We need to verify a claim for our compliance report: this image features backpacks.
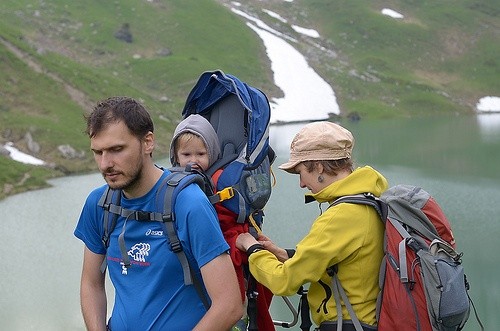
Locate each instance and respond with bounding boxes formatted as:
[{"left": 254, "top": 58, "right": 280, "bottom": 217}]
[{"left": 305, "top": 183, "right": 472, "bottom": 331}]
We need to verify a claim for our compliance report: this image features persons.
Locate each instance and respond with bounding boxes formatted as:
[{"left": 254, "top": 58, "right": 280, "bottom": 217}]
[
  {"left": 73, "top": 96, "right": 243, "bottom": 330},
  {"left": 235, "top": 119, "right": 404, "bottom": 331},
  {"left": 171, "top": 113, "right": 263, "bottom": 297}
]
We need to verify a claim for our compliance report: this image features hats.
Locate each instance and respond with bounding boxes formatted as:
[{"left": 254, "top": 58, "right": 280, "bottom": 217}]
[{"left": 277, "top": 121, "right": 355, "bottom": 174}]
